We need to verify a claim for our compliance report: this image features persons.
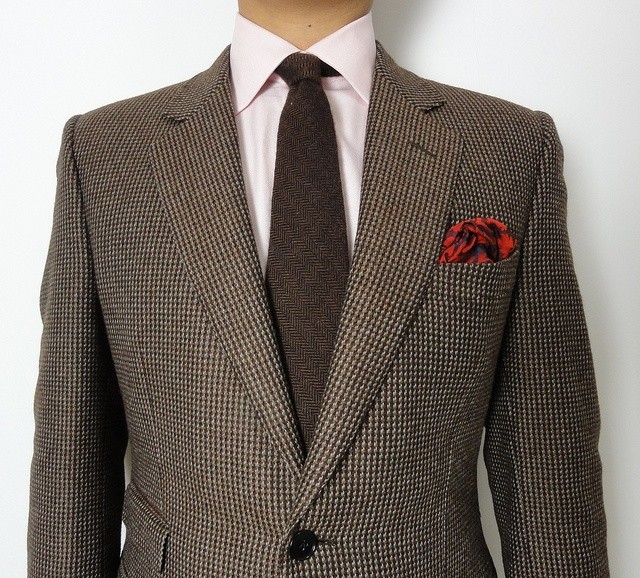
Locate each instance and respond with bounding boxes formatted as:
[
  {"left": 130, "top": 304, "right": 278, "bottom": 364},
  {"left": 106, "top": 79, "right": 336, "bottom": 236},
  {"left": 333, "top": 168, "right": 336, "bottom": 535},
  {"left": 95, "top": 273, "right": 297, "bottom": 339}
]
[{"left": 26, "top": 1, "right": 611, "bottom": 577}]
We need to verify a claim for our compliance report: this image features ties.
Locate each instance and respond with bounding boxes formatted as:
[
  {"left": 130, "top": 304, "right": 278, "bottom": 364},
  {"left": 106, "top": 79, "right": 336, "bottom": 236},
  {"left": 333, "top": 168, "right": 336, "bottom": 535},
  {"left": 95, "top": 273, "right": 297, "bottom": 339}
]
[{"left": 265, "top": 53, "right": 349, "bottom": 458}]
